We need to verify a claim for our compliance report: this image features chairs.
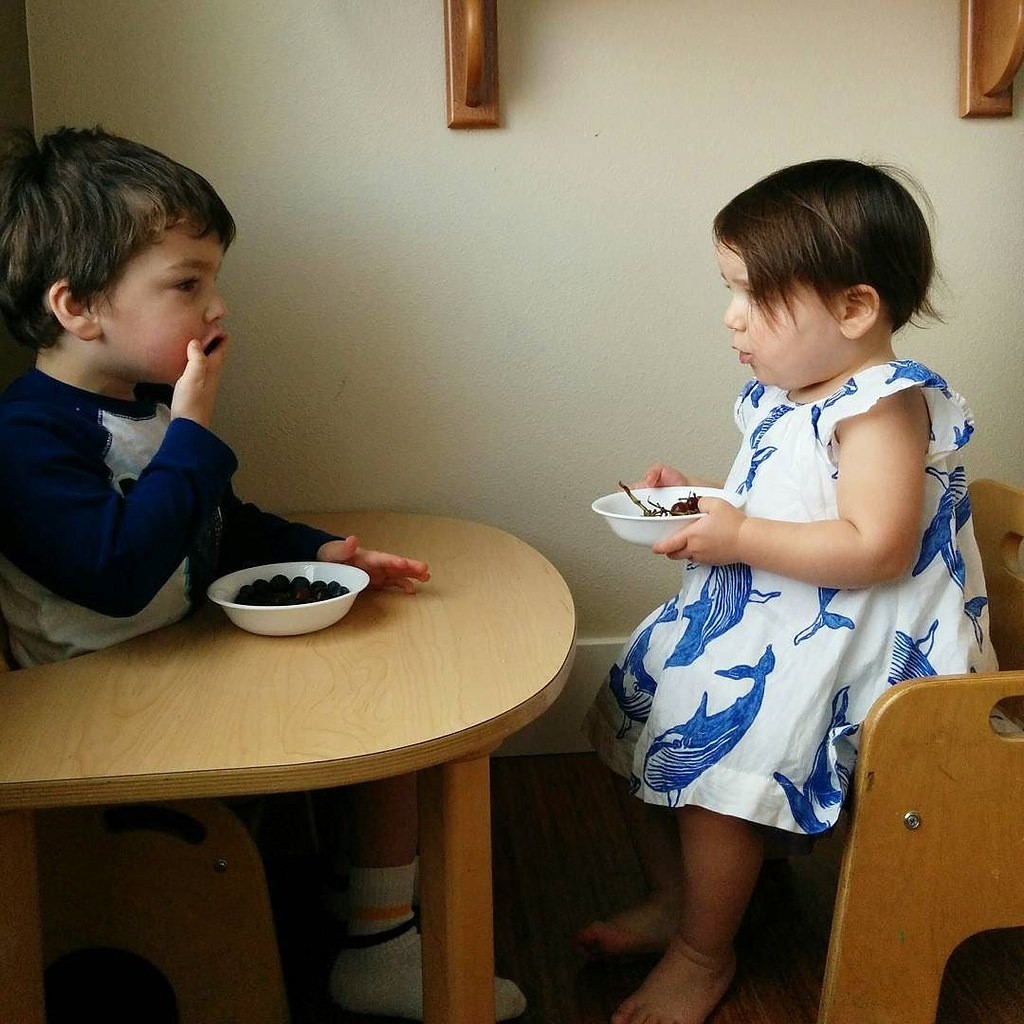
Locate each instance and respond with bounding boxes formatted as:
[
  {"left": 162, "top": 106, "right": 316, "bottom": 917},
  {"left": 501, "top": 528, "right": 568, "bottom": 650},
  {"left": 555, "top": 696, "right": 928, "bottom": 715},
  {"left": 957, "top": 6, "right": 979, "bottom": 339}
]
[{"left": 814, "top": 484, "right": 1023, "bottom": 1024}]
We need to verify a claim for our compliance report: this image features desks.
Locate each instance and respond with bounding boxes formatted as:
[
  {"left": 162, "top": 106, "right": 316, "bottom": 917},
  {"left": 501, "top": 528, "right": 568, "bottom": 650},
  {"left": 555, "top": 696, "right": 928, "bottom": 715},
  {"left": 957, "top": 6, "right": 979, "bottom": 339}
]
[{"left": 0, "top": 513, "right": 584, "bottom": 1024}]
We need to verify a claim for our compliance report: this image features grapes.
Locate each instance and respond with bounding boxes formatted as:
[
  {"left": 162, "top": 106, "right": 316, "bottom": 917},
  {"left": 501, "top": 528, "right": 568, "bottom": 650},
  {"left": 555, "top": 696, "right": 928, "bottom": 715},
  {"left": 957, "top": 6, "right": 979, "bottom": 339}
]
[
  {"left": 234, "top": 573, "right": 349, "bottom": 606},
  {"left": 670, "top": 497, "right": 699, "bottom": 516}
]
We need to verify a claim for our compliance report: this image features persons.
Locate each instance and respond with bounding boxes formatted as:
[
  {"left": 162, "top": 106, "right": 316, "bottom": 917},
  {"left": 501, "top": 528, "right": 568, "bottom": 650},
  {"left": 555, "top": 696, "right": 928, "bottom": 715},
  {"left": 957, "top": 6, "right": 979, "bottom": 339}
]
[
  {"left": 580, "top": 155, "right": 1002, "bottom": 1024},
  {"left": 0, "top": 121, "right": 527, "bottom": 1023}
]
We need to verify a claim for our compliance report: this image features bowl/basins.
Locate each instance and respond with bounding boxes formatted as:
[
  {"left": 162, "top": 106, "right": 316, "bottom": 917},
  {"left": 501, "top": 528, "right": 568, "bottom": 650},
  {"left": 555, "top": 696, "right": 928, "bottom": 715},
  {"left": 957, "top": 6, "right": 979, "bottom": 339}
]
[
  {"left": 590, "top": 486, "right": 747, "bottom": 546},
  {"left": 207, "top": 561, "right": 371, "bottom": 635}
]
